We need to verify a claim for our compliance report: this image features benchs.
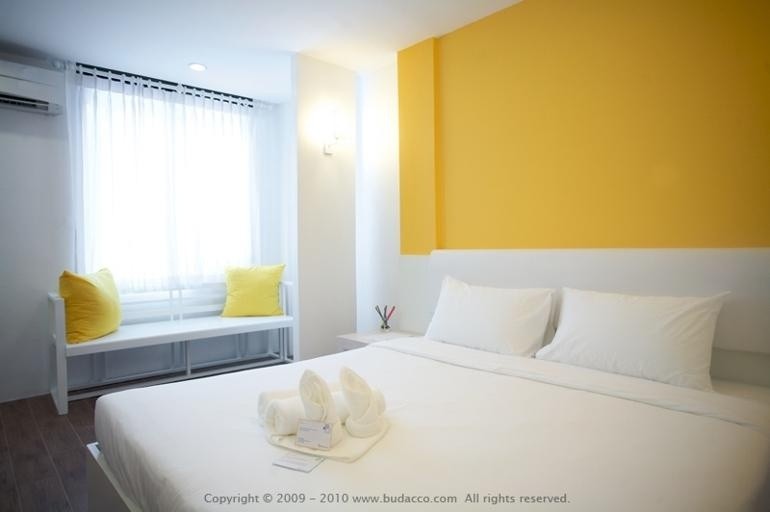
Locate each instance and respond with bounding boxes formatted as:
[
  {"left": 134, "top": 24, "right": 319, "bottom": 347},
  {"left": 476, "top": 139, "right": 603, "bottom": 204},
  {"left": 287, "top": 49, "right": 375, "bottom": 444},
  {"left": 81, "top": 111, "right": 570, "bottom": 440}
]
[{"left": 46, "top": 272, "right": 294, "bottom": 415}]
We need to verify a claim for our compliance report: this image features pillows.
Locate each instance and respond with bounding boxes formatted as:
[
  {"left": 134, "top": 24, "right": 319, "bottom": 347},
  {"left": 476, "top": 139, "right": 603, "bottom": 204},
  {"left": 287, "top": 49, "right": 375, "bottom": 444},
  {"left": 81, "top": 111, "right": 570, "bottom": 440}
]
[
  {"left": 219, "top": 262, "right": 284, "bottom": 317},
  {"left": 425, "top": 275, "right": 556, "bottom": 359},
  {"left": 537, "top": 286, "right": 730, "bottom": 397},
  {"left": 58, "top": 267, "right": 122, "bottom": 345}
]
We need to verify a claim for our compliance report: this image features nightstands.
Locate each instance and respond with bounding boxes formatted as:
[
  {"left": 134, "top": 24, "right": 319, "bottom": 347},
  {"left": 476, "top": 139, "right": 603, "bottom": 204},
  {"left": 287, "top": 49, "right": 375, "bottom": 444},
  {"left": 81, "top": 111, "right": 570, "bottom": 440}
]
[{"left": 335, "top": 330, "right": 413, "bottom": 356}]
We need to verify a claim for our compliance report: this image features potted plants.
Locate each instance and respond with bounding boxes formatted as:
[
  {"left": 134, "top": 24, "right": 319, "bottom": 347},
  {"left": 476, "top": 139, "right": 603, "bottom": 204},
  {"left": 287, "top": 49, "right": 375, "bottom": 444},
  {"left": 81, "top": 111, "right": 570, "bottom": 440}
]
[{"left": 373, "top": 304, "right": 395, "bottom": 337}]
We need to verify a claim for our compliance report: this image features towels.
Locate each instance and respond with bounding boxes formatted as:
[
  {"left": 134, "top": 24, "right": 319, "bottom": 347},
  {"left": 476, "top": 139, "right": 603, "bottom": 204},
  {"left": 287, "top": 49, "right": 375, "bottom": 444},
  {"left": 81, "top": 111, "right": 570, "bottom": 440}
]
[{"left": 252, "top": 355, "right": 396, "bottom": 469}]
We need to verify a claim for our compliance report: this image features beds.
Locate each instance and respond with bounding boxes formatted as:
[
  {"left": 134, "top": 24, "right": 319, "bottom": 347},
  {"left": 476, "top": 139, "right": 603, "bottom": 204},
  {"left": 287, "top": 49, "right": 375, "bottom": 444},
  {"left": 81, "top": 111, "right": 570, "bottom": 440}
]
[{"left": 79, "top": 242, "right": 770, "bottom": 512}]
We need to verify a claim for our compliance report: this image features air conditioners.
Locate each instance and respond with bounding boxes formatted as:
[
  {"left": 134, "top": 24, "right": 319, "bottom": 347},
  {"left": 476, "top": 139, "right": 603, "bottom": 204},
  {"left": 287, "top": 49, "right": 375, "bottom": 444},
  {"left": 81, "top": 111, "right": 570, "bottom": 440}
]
[{"left": 0, "top": 57, "right": 65, "bottom": 117}]
